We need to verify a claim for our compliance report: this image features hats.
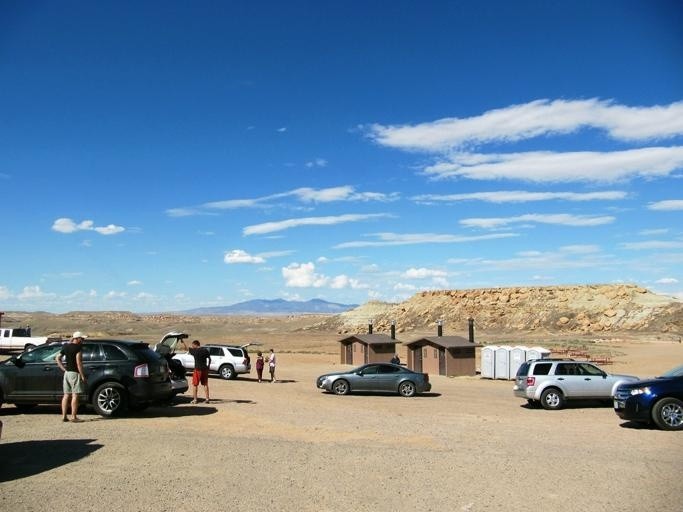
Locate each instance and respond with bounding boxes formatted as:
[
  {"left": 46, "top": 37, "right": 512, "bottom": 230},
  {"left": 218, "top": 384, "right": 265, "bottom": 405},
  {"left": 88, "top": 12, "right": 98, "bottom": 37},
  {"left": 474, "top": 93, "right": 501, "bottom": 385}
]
[{"left": 72, "top": 332, "right": 88, "bottom": 338}]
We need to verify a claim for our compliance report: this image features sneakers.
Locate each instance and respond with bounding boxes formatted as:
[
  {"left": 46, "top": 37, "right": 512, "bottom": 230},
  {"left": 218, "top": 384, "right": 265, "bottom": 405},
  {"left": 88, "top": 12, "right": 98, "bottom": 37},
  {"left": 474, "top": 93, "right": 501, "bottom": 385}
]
[
  {"left": 203, "top": 400, "right": 209, "bottom": 404},
  {"left": 191, "top": 400, "right": 198, "bottom": 404}
]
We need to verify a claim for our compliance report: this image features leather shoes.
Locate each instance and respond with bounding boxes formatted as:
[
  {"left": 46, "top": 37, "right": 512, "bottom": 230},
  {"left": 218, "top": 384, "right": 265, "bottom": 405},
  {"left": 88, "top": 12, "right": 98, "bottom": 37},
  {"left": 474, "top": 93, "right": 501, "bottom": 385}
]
[
  {"left": 63, "top": 418, "right": 70, "bottom": 422},
  {"left": 72, "top": 418, "right": 85, "bottom": 422}
]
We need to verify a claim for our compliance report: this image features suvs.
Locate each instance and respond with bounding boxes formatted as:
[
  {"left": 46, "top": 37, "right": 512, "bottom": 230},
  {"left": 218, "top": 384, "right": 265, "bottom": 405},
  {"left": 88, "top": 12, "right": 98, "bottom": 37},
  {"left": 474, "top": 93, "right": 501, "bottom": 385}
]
[
  {"left": 170, "top": 342, "right": 252, "bottom": 378},
  {"left": 616, "top": 365, "right": 683, "bottom": 429},
  {"left": 514, "top": 361, "right": 640, "bottom": 406}
]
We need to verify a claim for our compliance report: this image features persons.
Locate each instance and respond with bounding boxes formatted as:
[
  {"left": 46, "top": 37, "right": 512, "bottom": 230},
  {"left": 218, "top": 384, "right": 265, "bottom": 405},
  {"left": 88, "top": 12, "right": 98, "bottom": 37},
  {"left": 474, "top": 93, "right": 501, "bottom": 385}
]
[
  {"left": 179, "top": 335, "right": 212, "bottom": 405},
  {"left": 55, "top": 332, "right": 88, "bottom": 423},
  {"left": 255, "top": 350, "right": 265, "bottom": 381},
  {"left": 265, "top": 347, "right": 278, "bottom": 381},
  {"left": 25, "top": 322, "right": 32, "bottom": 336},
  {"left": 391, "top": 352, "right": 401, "bottom": 369}
]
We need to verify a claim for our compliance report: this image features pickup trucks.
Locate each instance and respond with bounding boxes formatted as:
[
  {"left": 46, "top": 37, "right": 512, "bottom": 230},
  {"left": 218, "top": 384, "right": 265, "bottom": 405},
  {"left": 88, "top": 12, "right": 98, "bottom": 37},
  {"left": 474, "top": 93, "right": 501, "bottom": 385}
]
[{"left": 0, "top": 328, "right": 47, "bottom": 351}]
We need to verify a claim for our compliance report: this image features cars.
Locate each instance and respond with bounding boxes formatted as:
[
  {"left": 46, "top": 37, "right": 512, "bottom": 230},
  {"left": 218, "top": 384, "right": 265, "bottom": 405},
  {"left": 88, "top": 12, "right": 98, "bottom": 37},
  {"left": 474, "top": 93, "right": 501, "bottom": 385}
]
[
  {"left": 150, "top": 332, "right": 188, "bottom": 394},
  {"left": 318, "top": 362, "right": 431, "bottom": 396},
  {"left": 0, "top": 340, "right": 171, "bottom": 416}
]
